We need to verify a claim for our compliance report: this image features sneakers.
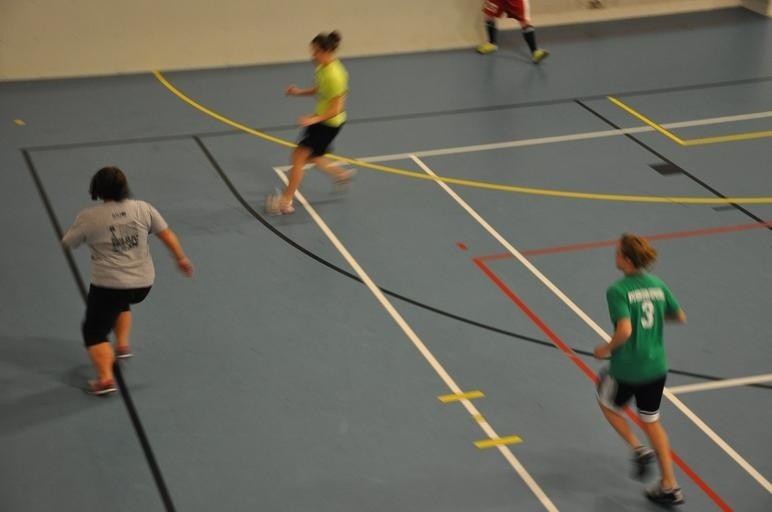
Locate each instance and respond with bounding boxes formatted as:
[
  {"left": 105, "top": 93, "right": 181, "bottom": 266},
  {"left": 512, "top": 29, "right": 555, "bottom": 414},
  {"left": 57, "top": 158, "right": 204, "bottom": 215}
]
[
  {"left": 477, "top": 42, "right": 498, "bottom": 54},
  {"left": 115, "top": 346, "right": 134, "bottom": 358},
  {"left": 266, "top": 201, "right": 294, "bottom": 215},
  {"left": 84, "top": 381, "right": 118, "bottom": 395},
  {"left": 631, "top": 445, "right": 684, "bottom": 505},
  {"left": 532, "top": 50, "right": 550, "bottom": 63}
]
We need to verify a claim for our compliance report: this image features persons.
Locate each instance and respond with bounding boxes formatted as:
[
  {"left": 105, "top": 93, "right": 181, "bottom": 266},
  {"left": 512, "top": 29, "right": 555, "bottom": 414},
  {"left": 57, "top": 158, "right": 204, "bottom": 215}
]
[
  {"left": 593, "top": 233, "right": 687, "bottom": 507},
  {"left": 62, "top": 166, "right": 193, "bottom": 394},
  {"left": 265, "top": 29, "right": 356, "bottom": 214},
  {"left": 476, "top": 0, "right": 551, "bottom": 63}
]
